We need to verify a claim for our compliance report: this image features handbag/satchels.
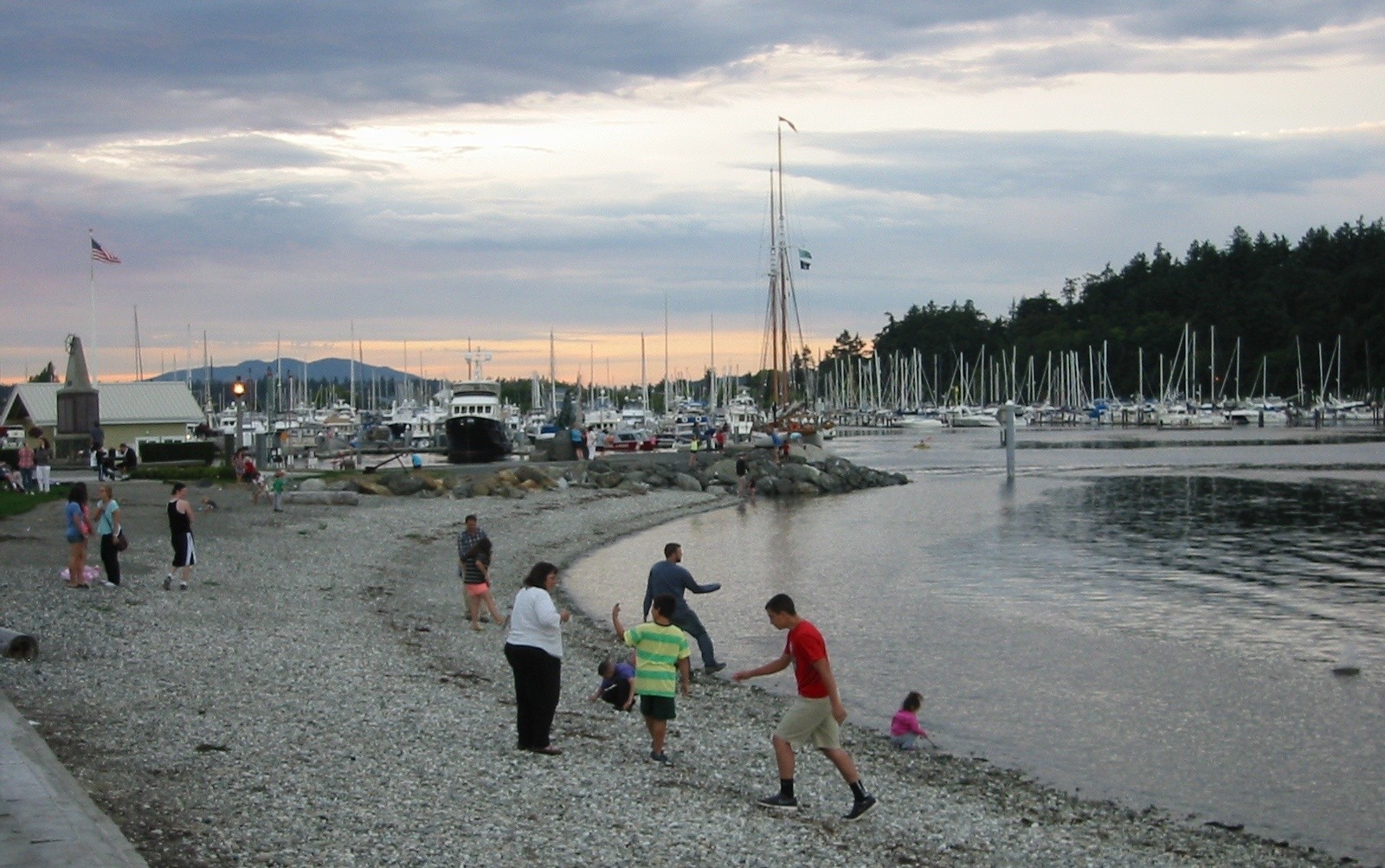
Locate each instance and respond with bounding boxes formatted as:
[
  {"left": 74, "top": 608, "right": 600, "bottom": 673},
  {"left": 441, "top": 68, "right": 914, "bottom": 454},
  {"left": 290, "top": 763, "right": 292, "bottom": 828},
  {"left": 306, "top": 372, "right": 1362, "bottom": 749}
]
[{"left": 116, "top": 533, "right": 127, "bottom": 551}]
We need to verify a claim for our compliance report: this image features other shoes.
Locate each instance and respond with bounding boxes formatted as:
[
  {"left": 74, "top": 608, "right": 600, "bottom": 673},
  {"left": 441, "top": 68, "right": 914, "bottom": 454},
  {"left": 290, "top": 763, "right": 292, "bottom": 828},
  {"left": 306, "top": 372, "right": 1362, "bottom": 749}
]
[
  {"left": 99, "top": 580, "right": 106, "bottom": 584},
  {"left": 650, "top": 750, "right": 668, "bottom": 764},
  {"left": 757, "top": 794, "right": 798, "bottom": 811},
  {"left": 180, "top": 581, "right": 187, "bottom": 589},
  {"left": 69, "top": 583, "right": 80, "bottom": 590},
  {"left": 517, "top": 742, "right": 528, "bottom": 750},
  {"left": 840, "top": 793, "right": 879, "bottom": 823},
  {"left": 705, "top": 661, "right": 727, "bottom": 675},
  {"left": 80, "top": 583, "right": 90, "bottom": 590},
  {"left": 164, "top": 576, "right": 171, "bottom": 591},
  {"left": 531, "top": 745, "right": 562, "bottom": 756},
  {"left": 104, "top": 582, "right": 116, "bottom": 587}
]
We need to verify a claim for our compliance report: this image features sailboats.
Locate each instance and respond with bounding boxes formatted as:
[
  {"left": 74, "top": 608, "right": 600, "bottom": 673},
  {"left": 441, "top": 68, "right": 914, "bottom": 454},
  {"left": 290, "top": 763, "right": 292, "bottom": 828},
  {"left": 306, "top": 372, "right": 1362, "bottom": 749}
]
[{"left": 189, "top": 116, "right": 1385, "bottom": 457}]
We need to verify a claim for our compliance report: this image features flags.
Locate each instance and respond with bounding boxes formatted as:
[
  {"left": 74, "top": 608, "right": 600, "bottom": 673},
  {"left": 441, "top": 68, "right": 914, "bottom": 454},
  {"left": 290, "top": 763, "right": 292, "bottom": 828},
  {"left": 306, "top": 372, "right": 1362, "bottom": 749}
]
[{"left": 91, "top": 237, "right": 120, "bottom": 265}]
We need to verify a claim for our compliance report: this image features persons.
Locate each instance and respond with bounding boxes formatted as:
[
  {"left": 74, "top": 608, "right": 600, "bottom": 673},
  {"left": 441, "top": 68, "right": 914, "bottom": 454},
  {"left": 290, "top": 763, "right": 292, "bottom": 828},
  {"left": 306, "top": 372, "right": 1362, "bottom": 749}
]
[
  {"left": 231, "top": 446, "right": 285, "bottom": 512},
  {"left": 504, "top": 561, "right": 571, "bottom": 755},
  {"left": 643, "top": 543, "right": 728, "bottom": 675},
  {"left": 456, "top": 515, "right": 505, "bottom": 631},
  {"left": 0, "top": 441, "right": 34, "bottom": 495},
  {"left": 571, "top": 422, "right": 608, "bottom": 461},
  {"left": 93, "top": 485, "right": 123, "bottom": 586},
  {"left": 163, "top": 483, "right": 196, "bottom": 590},
  {"left": 411, "top": 453, "right": 421, "bottom": 470},
  {"left": 34, "top": 438, "right": 53, "bottom": 493},
  {"left": 90, "top": 420, "right": 107, "bottom": 482},
  {"left": 64, "top": 481, "right": 91, "bottom": 590},
  {"left": 733, "top": 594, "right": 879, "bottom": 823},
  {"left": 767, "top": 429, "right": 790, "bottom": 464},
  {"left": 116, "top": 443, "right": 137, "bottom": 480},
  {"left": 690, "top": 429, "right": 724, "bottom": 454},
  {"left": 736, "top": 456, "right": 750, "bottom": 491},
  {"left": 612, "top": 593, "right": 692, "bottom": 763},
  {"left": 890, "top": 692, "right": 928, "bottom": 749},
  {"left": 591, "top": 662, "right": 639, "bottom": 713}
]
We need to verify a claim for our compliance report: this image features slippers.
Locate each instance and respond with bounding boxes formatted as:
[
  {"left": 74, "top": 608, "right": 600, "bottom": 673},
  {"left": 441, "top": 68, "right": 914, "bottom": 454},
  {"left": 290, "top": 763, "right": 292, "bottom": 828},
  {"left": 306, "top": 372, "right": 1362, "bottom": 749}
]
[
  {"left": 472, "top": 627, "right": 485, "bottom": 631},
  {"left": 500, "top": 614, "right": 510, "bottom": 630}
]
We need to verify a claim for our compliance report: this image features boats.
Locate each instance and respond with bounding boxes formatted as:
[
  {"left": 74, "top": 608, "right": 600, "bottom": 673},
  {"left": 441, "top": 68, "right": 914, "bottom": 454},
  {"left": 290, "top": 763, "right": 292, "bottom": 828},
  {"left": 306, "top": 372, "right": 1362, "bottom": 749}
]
[{"left": 443, "top": 346, "right": 517, "bottom": 464}]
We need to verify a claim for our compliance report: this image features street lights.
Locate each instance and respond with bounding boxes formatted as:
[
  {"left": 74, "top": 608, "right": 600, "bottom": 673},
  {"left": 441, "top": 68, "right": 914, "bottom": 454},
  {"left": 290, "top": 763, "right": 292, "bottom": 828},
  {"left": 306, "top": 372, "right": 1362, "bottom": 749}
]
[{"left": 233, "top": 376, "right": 246, "bottom": 454}]
